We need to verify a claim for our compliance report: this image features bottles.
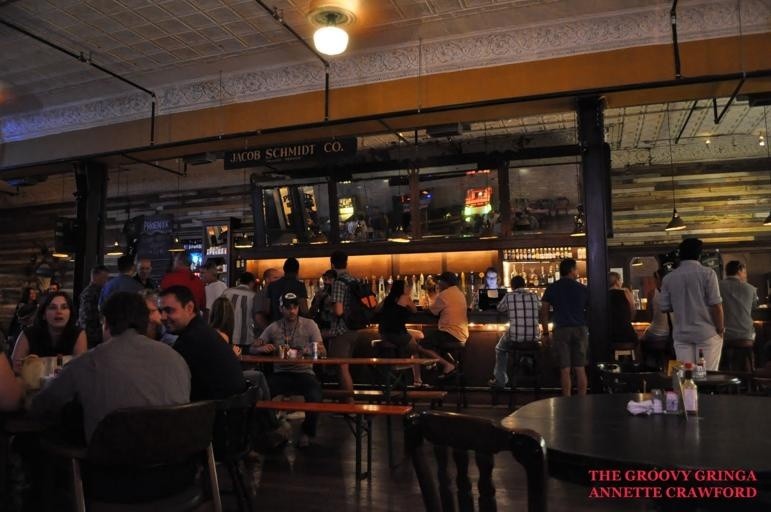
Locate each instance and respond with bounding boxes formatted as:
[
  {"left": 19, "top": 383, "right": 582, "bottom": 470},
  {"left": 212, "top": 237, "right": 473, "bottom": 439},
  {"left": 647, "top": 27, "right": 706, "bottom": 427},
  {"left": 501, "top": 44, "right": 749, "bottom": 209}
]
[
  {"left": 418, "top": 288, "right": 426, "bottom": 305},
  {"left": 312, "top": 342, "right": 318, "bottom": 360},
  {"left": 650, "top": 389, "right": 663, "bottom": 413},
  {"left": 503, "top": 247, "right": 574, "bottom": 261},
  {"left": 632, "top": 289, "right": 640, "bottom": 310},
  {"left": 303, "top": 268, "right": 485, "bottom": 296},
  {"left": 696, "top": 348, "right": 706, "bottom": 379},
  {"left": 682, "top": 362, "right": 698, "bottom": 415},
  {"left": 52, "top": 353, "right": 63, "bottom": 378},
  {"left": 510, "top": 264, "right": 561, "bottom": 288}
]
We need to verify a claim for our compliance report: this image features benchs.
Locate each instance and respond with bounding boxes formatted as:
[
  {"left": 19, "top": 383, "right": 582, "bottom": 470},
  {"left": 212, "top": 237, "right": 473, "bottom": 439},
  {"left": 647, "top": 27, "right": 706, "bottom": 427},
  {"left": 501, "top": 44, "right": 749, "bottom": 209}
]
[
  {"left": 303, "top": 388, "right": 450, "bottom": 408},
  {"left": 254, "top": 397, "right": 415, "bottom": 489}
]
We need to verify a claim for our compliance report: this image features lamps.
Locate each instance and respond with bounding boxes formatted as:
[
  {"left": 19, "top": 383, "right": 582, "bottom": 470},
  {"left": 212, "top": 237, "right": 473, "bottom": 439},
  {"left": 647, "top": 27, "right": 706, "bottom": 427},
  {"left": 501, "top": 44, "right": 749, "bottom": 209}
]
[
  {"left": 306, "top": 7, "right": 355, "bottom": 57},
  {"left": 45, "top": 110, "right": 689, "bottom": 268},
  {"left": 761, "top": 107, "right": 771, "bottom": 226}
]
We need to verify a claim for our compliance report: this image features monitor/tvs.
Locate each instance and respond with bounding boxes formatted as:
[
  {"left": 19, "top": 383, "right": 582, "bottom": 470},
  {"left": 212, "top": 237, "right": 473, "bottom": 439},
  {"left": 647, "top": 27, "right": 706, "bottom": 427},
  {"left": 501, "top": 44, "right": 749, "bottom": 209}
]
[{"left": 478, "top": 288, "right": 506, "bottom": 308}]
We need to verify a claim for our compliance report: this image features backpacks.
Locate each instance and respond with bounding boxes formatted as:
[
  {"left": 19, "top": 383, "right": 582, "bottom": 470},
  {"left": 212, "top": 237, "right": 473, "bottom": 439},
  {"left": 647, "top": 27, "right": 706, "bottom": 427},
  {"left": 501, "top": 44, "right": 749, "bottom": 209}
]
[{"left": 334, "top": 277, "right": 377, "bottom": 330}]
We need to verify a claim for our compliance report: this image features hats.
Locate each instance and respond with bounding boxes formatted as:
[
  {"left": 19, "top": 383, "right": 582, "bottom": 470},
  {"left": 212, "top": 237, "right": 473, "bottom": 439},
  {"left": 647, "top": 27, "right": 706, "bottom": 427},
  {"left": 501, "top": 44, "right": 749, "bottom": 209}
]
[
  {"left": 434, "top": 271, "right": 460, "bottom": 285},
  {"left": 279, "top": 290, "right": 302, "bottom": 306}
]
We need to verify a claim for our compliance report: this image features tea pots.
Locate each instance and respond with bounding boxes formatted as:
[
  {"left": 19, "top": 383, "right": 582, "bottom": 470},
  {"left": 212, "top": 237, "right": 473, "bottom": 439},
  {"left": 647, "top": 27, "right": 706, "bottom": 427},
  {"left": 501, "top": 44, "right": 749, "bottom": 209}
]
[{"left": 14, "top": 353, "right": 49, "bottom": 390}]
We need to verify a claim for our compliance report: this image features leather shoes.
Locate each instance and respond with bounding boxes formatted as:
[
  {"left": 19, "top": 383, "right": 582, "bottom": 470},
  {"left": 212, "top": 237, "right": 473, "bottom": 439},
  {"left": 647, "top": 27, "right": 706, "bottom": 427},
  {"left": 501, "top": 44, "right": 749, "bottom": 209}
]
[
  {"left": 414, "top": 381, "right": 435, "bottom": 389},
  {"left": 438, "top": 362, "right": 459, "bottom": 379}
]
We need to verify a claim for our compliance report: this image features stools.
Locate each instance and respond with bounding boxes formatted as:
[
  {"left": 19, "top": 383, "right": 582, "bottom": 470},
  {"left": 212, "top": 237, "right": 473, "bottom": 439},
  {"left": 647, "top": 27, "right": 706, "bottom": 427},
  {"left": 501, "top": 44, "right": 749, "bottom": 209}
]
[
  {"left": 727, "top": 337, "right": 757, "bottom": 378},
  {"left": 613, "top": 342, "right": 642, "bottom": 376},
  {"left": 371, "top": 337, "right": 406, "bottom": 387},
  {"left": 506, "top": 345, "right": 543, "bottom": 416},
  {"left": 431, "top": 339, "right": 469, "bottom": 413}
]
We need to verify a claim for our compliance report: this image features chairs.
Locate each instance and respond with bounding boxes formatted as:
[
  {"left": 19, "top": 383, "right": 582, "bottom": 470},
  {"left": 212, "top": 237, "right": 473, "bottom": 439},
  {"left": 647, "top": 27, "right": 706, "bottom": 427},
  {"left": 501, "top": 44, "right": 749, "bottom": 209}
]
[
  {"left": 603, "top": 371, "right": 658, "bottom": 398},
  {"left": 598, "top": 360, "right": 634, "bottom": 397},
  {"left": 39, "top": 401, "right": 223, "bottom": 510},
  {"left": 210, "top": 368, "right": 271, "bottom": 510},
  {"left": 408, "top": 410, "right": 549, "bottom": 511}
]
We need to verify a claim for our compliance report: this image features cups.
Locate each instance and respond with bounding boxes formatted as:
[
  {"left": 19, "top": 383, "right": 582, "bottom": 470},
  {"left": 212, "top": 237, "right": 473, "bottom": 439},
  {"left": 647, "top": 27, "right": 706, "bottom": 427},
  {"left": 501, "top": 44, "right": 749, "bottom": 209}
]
[
  {"left": 39, "top": 377, "right": 54, "bottom": 392},
  {"left": 287, "top": 350, "right": 297, "bottom": 360},
  {"left": 573, "top": 215, "right": 585, "bottom": 232},
  {"left": 665, "top": 392, "right": 679, "bottom": 412},
  {"left": 633, "top": 392, "right": 650, "bottom": 403},
  {"left": 641, "top": 298, "right": 647, "bottom": 309}
]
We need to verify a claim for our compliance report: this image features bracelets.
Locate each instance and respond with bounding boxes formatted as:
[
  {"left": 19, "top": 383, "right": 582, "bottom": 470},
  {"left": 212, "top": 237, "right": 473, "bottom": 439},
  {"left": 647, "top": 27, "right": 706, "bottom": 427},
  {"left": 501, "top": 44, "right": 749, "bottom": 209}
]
[{"left": 542, "top": 331, "right": 550, "bottom": 337}]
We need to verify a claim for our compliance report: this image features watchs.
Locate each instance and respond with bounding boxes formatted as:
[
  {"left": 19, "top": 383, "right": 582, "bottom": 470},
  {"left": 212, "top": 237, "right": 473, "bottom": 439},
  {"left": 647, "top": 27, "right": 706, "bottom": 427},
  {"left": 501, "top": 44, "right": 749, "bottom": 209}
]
[{"left": 717, "top": 328, "right": 725, "bottom": 333}]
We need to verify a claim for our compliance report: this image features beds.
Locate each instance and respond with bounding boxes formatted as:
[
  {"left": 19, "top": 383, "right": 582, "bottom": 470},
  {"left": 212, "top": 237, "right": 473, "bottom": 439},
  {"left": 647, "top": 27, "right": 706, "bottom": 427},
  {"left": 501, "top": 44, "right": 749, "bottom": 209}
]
[{"left": 237, "top": 352, "right": 441, "bottom": 401}]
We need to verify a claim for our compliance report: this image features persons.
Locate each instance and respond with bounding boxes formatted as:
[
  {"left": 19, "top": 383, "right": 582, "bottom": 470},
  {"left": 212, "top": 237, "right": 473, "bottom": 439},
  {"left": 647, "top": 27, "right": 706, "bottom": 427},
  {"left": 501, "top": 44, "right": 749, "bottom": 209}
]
[
  {"left": 487, "top": 275, "right": 540, "bottom": 386},
  {"left": 718, "top": 260, "right": 758, "bottom": 368},
  {"left": 461, "top": 204, "right": 538, "bottom": 232},
  {"left": 658, "top": 239, "right": 726, "bottom": 372},
  {"left": 303, "top": 206, "right": 388, "bottom": 241},
  {"left": 470, "top": 267, "right": 504, "bottom": 312},
  {"left": 540, "top": 257, "right": 589, "bottom": 397},
  {"left": 641, "top": 269, "right": 673, "bottom": 371},
  {"left": 608, "top": 271, "right": 639, "bottom": 383}
]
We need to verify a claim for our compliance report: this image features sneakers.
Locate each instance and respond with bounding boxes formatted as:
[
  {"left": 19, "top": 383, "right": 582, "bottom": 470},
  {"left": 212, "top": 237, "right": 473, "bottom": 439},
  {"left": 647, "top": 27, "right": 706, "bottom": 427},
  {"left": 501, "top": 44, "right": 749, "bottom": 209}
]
[{"left": 488, "top": 378, "right": 505, "bottom": 392}]
